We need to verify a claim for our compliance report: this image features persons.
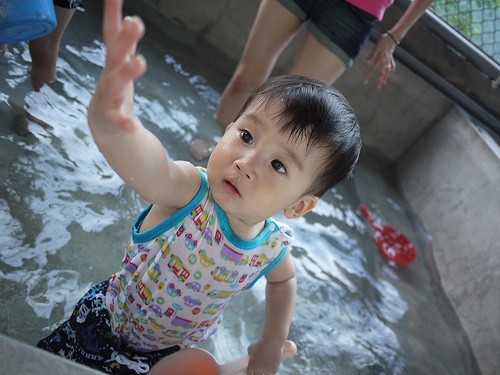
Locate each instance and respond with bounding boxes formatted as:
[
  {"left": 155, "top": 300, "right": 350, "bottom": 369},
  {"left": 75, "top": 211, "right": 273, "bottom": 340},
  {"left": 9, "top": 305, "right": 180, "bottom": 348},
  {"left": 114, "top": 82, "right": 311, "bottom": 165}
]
[
  {"left": 32, "top": 0, "right": 363, "bottom": 374},
  {"left": 0, "top": 0, "right": 80, "bottom": 139},
  {"left": 190, "top": 0, "right": 431, "bottom": 160}
]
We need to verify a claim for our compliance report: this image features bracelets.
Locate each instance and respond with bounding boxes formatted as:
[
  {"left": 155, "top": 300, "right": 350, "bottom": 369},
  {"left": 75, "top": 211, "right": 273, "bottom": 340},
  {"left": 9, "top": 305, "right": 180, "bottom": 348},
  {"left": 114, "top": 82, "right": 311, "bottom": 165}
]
[{"left": 386, "top": 30, "right": 400, "bottom": 45}]
